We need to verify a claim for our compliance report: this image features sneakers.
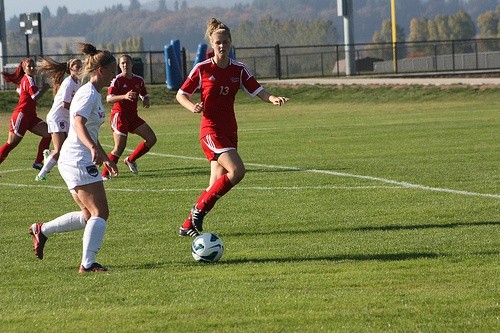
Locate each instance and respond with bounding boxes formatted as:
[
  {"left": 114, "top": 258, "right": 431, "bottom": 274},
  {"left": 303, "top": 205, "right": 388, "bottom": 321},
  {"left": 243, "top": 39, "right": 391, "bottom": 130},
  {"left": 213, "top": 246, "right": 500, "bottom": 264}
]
[
  {"left": 43, "top": 150, "right": 50, "bottom": 161},
  {"left": 191, "top": 203, "right": 207, "bottom": 233},
  {"left": 178, "top": 226, "right": 201, "bottom": 237},
  {"left": 33, "top": 162, "right": 50, "bottom": 173},
  {"left": 34, "top": 175, "right": 47, "bottom": 182},
  {"left": 123, "top": 156, "right": 138, "bottom": 173},
  {"left": 27, "top": 223, "right": 49, "bottom": 260},
  {"left": 79, "top": 262, "right": 106, "bottom": 272},
  {"left": 102, "top": 176, "right": 111, "bottom": 180}
]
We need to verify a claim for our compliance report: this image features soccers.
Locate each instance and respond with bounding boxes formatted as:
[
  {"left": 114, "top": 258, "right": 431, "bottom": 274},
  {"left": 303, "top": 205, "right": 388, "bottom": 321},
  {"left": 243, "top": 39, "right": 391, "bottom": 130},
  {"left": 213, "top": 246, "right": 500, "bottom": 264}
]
[{"left": 191, "top": 231, "right": 225, "bottom": 263}]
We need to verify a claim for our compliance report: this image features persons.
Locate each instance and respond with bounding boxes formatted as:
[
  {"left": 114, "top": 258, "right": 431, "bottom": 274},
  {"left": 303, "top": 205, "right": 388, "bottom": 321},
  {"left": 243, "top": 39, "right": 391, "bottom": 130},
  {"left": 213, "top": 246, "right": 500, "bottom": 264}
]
[
  {"left": 175, "top": 18, "right": 290, "bottom": 237},
  {"left": 35, "top": 55, "right": 83, "bottom": 181},
  {"left": 0, "top": 58, "right": 51, "bottom": 173},
  {"left": 99, "top": 55, "right": 157, "bottom": 180},
  {"left": 29, "top": 44, "right": 117, "bottom": 273}
]
